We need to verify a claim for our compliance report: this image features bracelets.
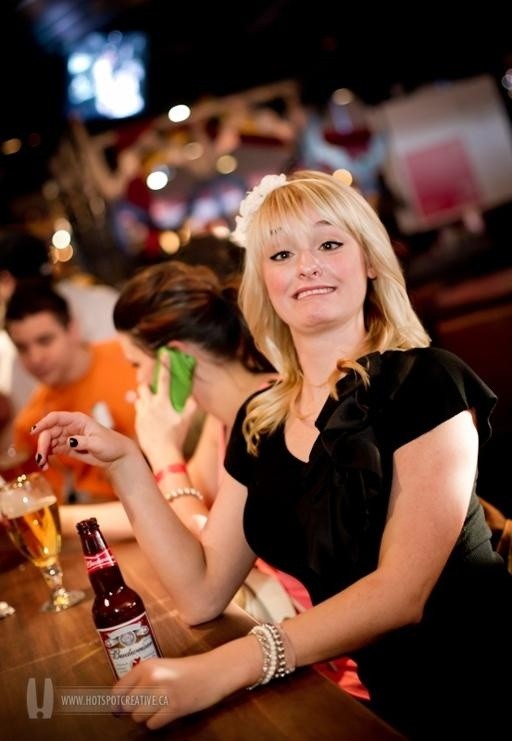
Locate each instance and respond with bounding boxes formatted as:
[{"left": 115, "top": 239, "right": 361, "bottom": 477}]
[
  {"left": 245, "top": 619, "right": 299, "bottom": 692},
  {"left": 161, "top": 487, "right": 206, "bottom": 505}
]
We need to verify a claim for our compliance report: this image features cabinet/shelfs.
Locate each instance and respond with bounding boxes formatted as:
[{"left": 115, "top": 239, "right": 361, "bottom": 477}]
[{"left": 0, "top": 534, "right": 408, "bottom": 741}]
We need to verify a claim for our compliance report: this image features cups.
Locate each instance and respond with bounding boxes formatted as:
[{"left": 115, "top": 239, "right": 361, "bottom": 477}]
[
  {"left": 0, "top": 470, "right": 86, "bottom": 615},
  {"left": 0, "top": 452, "right": 40, "bottom": 483}
]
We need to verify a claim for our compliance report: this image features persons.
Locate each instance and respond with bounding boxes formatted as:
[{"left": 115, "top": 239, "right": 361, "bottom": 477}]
[
  {"left": 28, "top": 170, "right": 512, "bottom": 741},
  {"left": 112, "top": 261, "right": 374, "bottom": 702},
  {"left": 2, "top": 281, "right": 137, "bottom": 505}
]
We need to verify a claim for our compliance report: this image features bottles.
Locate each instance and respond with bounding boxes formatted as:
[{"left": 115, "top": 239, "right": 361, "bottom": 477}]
[{"left": 76, "top": 517, "right": 163, "bottom": 682}]
